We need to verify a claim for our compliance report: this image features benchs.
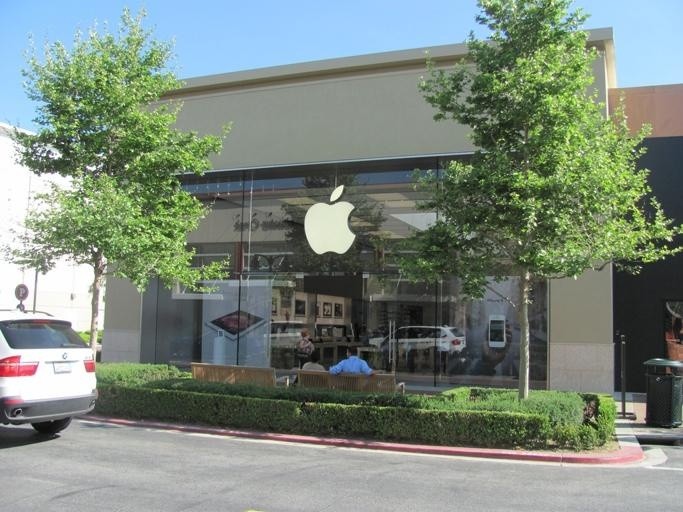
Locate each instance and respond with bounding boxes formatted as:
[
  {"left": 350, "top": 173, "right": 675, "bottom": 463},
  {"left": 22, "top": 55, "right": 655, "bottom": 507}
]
[
  {"left": 297, "top": 368, "right": 405, "bottom": 399},
  {"left": 188, "top": 361, "right": 288, "bottom": 391}
]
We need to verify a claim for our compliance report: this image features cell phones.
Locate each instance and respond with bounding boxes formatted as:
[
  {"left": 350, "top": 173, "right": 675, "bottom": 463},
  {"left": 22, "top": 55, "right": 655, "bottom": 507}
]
[{"left": 489, "top": 315, "right": 506, "bottom": 348}]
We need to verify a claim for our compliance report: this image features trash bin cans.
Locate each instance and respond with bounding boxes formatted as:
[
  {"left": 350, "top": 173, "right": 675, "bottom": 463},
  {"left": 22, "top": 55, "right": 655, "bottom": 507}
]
[{"left": 643, "top": 358, "right": 683, "bottom": 428}]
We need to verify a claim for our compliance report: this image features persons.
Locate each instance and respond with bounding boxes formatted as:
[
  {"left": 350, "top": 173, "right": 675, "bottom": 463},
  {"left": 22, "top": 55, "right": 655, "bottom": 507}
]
[
  {"left": 296, "top": 331, "right": 315, "bottom": 356},
  {"left": 301, "top": 351, "right": 326, "bottom": 371},
  {"left": 447, "top": 317, "right": 512, "bottom": 376},
  {"left": 329, "top": 347, "right": 377, "bottom": 376}
]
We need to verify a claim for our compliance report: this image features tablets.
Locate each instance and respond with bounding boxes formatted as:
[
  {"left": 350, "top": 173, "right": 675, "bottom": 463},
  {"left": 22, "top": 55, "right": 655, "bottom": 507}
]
[{"left": 206, "top": 309, "right": 267, "bottom": 340}]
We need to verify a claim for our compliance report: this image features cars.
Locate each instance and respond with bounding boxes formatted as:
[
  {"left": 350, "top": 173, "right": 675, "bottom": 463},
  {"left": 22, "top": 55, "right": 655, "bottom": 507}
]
[
  {"left": 367, "top": 324, "right": 466, "bottom": 364},
  {"left": 0, "top": 308, "right": 99, "bottom": 435},
  {"left": 263, "top": 321, "right": 317, "bottom": 351}
]
